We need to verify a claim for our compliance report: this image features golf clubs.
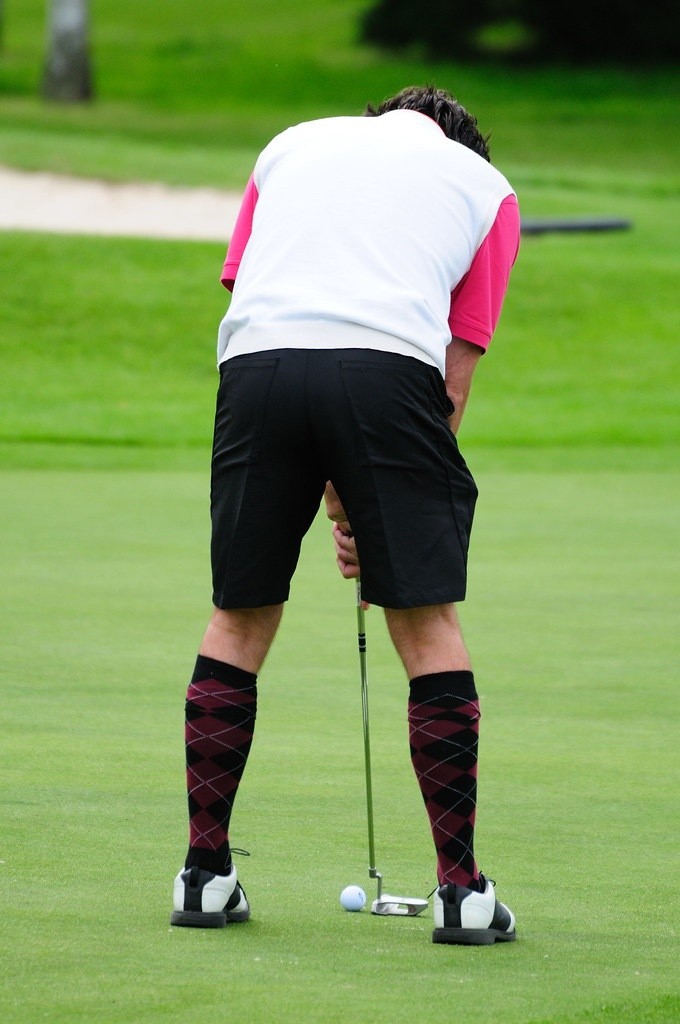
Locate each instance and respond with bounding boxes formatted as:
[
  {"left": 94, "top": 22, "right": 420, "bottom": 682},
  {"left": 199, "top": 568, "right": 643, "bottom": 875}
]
[{"left": 354, "top": 575, "right": 429, "bottom": 917}]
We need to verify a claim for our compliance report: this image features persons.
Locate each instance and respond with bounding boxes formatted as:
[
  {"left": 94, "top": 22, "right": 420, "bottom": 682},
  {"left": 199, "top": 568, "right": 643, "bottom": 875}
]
[{"left": 169, "top": 82, "right": 520, "bottom": 945}]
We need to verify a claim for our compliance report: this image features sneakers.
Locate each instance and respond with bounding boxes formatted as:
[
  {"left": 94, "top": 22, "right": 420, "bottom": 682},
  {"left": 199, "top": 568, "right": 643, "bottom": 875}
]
[
  {"left": 432, "top": 871, "right": 516, "bottom": 945},
  {"left": 171, "top": 848, "right": 250, "bottom": 928}
]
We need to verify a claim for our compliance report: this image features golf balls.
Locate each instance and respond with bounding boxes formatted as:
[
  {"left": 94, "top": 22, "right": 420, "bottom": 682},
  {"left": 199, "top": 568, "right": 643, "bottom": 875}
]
[{"left": 340, "top": 885, "right": 368, "bottom": 913}]
